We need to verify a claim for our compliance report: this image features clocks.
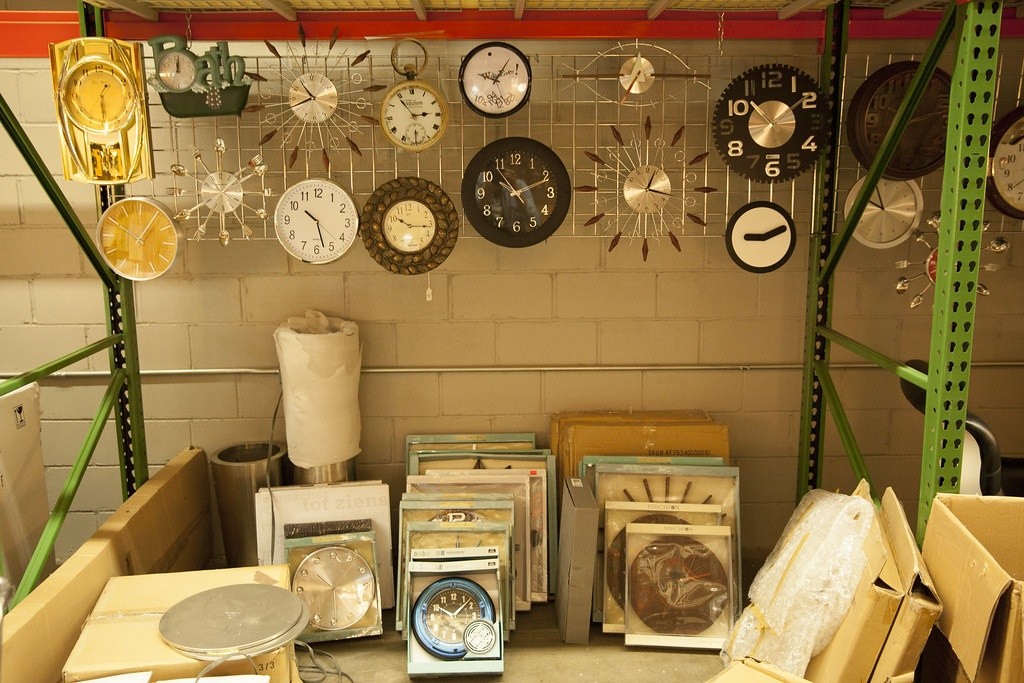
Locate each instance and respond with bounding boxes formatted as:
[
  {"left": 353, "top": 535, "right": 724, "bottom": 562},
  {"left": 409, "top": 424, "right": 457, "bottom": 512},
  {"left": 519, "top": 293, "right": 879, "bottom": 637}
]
[
  {"left": 428, "top": 509, "right": 490, "bottom": 522},
  {"left": 96, "top": 197, "right": 184, "bottom": 282},
  {"left": 573, "top": 115, "right": 717, "bottom": 262},
  {"left": 165, "top": 136, "right": 278, "bottom": 247},
  {"left": 147, "top": 36, "right": 251, "bottom": 121},
  {"left": 892, "top": 209, "right": 1010, "bottom": 310},
  {"left": 47, "top": 36, "right": 155, "bottom": 186},
  {"left": 411, "top": 577, "right": 498, "bottom": 661},
  {"left": 238, "top": 20, "right": 387, "bottom": 175},
  {"left": 418, "top": 532, "right": 505, "bottom": 548},
  {"left": 712, "top": 63, "right": 828, "bottom": 184},
  {"left": 459, "top": 42, "right": 532, "bottom": 118},
  {"left": 985, "top": 106, "right": 1024, "bottom": 222},
  {"left": 604, "top": 514, "right": 694, "bottom": 612},
  {"left": 378, "top": 38, "right": 450, "bottom": 153},
  {"left": 724, "top": 200, "right": 797, "bottom": 274},
  {"left": 273, "top": 177, "right": 360, "bottom": 264},
  {"left": 845, "top": 60, "right": 953, "bottom": 180},
  {"left": 622, "top": 477, "right": 714, "bottom": 503},
  {"left": 358, "top": 177, "right": 459, "bottom": 276},
  {"left": 626, "top": 534, "right": 730, "bottom": 636},
  {"left": 559, "top": 36, "right": 712, "bottom": 111},
  {"left": 842, "top": 175, "right": 924, "bottom": 250},
  {"left": 288, "top": 544, "right": 376, "bottom": 632},
  {"left": 461, "top": 136, "right": 571, "bottom": 248}
]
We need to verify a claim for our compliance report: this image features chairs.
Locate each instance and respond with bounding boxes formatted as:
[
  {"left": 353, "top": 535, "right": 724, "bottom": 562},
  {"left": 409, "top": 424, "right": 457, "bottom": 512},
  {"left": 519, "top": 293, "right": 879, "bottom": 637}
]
[{"left": 901, "top": 358, "right": 1024, "bottom": 498}]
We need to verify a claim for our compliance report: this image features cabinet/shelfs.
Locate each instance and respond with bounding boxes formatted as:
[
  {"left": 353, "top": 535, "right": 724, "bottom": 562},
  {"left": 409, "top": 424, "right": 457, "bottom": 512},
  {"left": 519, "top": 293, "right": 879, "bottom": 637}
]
[{"left": 0, "top": 0, "right": 1006, "bottom": 683}]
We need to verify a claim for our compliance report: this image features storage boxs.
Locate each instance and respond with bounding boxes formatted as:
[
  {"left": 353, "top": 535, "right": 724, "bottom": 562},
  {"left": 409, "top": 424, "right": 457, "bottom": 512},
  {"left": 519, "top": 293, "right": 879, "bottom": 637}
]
[
  {"left": 602, "top": 499, "right": 722, "bottom": 633},
  {"left": 591, "top": 462, "right": 743, "bottom": 624},
  {"left": 865, "top": 485, "right": 943, "bottom": 683},
  {"left": 55, "top": 563, "right": 306, "bottom": 683},
  {"left": 409, "top": 454, "right": 556, "bottom": 594},
  {"left": 555, "top": 474, "right": 600, "bottom": 646},
  {"left": 90, "top": 444, "right": 214, "bottom": 574},
  {"left": 401, "top": 492, "right": 515, "bottom": 501},
  {"left": 0, "top": 378, "right": 58, "bottom": 608},
  {"left": 559, "top": 418, "right": 730, "bottom": 515},
  {"left": 410, "top": 545, "right": 501, "bottom": 562},
  {"left": 581, "top": 453, "right": 723, "bottom": 501},
  {"left": 406, "top": 475, "right": 533, "bottom": 612},
  {"left": 283, "top": 531, "right": 385, "bottom": 646},
  {"left": 402, "top": 521, "right": 512, "bottom": 642},
  {"left": 551, "top": 409, "right": 714, "bottom": 502},
  {"left": 254, "top": 482, "right": 394, "bottom": 610},
  {"left": 623, "top": 522, "right": 735, "bottom": 650},
  {"left": 423, "top": 468, "right": 550, "bottom": 604},
  {"left": 407, "top": 559, "right": 504, "bottom": 679},
  {"left": 405, "top": 448, "right": 552, "bottom": 456},
  {"left": 407, "top": 434, "right": 536, "bottom": 447},
  {"left": 258, "top": 479, "right": 383, "bottom": 493},
  {"left": 922, "top": 492, "right": 1024, "bottom": 683},
  {"left": 703, "top": 656, "right": 819, "bottom": 683},
  {"left": 394, "top": 499, "right": 516, "bottom": 632},
  {"left": 0, "top": 518, "right": 131, "bottom": 683},
  {"left": 805, "top": 476, "right": 903, "bottom": 682}
]
